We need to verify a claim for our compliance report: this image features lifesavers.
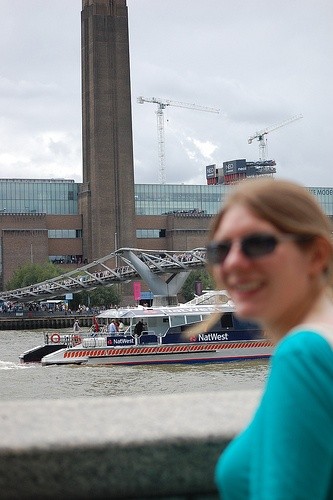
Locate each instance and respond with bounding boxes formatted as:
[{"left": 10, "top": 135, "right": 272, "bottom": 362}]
[
  {"left": 71, "top": 337, "right": 81, "bottom": 347},
  {"left": 51, "top": 333, "right": 61, "bottom": 343}
]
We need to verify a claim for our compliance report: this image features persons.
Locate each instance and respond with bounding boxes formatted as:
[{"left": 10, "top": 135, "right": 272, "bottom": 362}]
[
  {"left": 206, "top": 178, "right": 333, "bottom": 500},
  {"left": 0, "top": 301, "right": 145, "bottom": 335}
]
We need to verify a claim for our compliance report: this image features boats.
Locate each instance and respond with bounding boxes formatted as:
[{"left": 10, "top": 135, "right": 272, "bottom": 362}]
[{"left": 40, "top": 288, "right": 275, "bottom": 366}]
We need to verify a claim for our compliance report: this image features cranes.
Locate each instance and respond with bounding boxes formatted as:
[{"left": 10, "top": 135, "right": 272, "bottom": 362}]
[{"left": 135, "top": 96, "right": 221, "bottom": 184}]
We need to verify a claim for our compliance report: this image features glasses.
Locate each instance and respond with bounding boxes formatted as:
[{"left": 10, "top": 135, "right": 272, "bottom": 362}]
[{"left": 206, "top": 232, "right": 295, "bottom": 264}]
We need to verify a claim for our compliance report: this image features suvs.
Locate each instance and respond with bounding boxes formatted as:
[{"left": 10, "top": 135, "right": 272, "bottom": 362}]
[{"left": 248, "top": 116, "right": 304, "bottom": 160}]
